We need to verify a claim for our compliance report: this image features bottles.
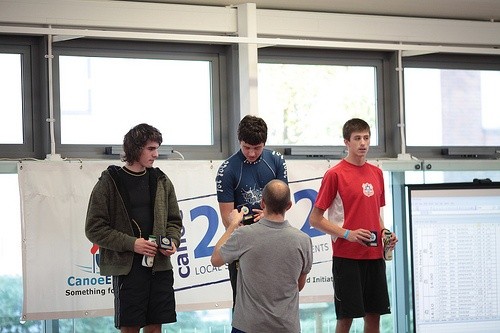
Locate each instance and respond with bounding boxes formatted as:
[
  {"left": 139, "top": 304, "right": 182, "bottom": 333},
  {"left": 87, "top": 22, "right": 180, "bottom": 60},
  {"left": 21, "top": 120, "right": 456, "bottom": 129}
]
[
  {"left": 382, "top": 231, "right": 393, "bottom": 261},
  {"left": 142, "top": 234, "right": 158, "bottom": 268}
]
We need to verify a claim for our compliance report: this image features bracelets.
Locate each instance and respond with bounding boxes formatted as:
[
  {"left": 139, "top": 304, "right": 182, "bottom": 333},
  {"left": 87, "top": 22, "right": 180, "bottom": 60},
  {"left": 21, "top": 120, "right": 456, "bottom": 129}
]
[
  {"left": 344, "top": 230, "right": 350, "bottom": 239},
  {"left": 381, "top": 228, "right": 390, "bottom": 232}
]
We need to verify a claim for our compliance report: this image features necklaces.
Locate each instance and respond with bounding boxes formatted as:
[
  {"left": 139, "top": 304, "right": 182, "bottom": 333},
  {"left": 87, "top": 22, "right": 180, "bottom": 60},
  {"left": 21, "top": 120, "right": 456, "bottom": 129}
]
[{"left": 121, "top": 166, "right": 146, "bottom": 176}]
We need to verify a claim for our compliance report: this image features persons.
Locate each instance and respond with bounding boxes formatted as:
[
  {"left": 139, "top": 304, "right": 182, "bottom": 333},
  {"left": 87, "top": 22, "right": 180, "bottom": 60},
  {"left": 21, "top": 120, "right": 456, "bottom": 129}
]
[
  {"left": 86, "top": 123, "right": 184, "bottom": 333},
  {"left": 309, "top": 117, "right": 398, "bottom": 333},
  {"left": 214, "top": 115, "right": 289, "bottom": 312},
  {"left": 211, "top": 179, "right": 314, "bottom": 333}
]
